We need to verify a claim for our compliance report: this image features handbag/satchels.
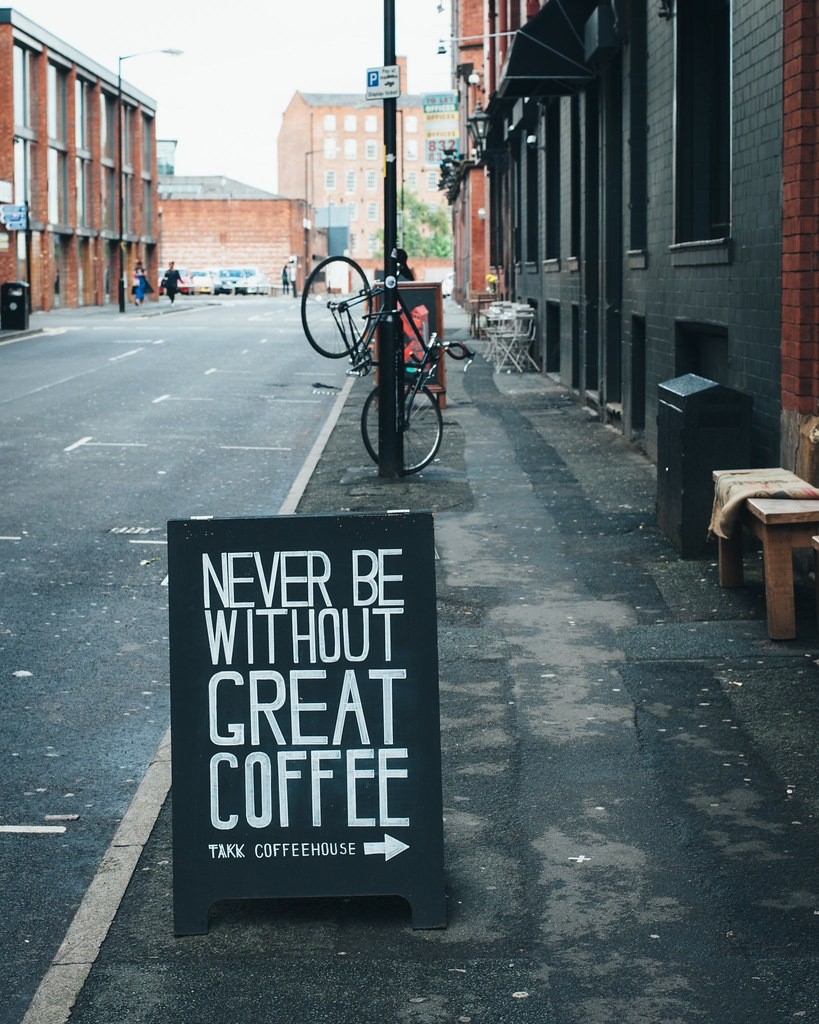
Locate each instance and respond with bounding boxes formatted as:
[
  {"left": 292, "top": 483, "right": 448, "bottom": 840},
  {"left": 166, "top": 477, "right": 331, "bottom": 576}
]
[
  {"left": 133, "top": 279, "right": 140, "bottom": 287},
  {"left": 161, "top": 279, "right": 168, "bottom": 288}
]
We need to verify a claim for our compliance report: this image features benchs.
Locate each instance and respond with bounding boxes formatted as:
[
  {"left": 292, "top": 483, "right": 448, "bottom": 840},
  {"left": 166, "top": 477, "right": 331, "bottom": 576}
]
[{"left": 705, "top": 468, "right": 819, "bottom": 639}]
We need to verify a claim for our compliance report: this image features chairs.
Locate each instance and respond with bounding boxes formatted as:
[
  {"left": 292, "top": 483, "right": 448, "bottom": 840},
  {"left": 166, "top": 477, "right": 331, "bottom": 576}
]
[
  {"left": 483, "top": 301, "right": 541, "bottom": 374},
  {"left": 470, "top": 293, "right": 497, "bottom": 340}
]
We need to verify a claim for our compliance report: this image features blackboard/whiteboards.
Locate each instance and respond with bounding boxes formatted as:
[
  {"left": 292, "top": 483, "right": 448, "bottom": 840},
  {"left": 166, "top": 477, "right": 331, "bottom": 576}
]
[
  {"left": 375, "top": 281, "right": 448, "bottom": 392},
  {"left": 162, "top": 507, "right": 453, "bottom": 932}
]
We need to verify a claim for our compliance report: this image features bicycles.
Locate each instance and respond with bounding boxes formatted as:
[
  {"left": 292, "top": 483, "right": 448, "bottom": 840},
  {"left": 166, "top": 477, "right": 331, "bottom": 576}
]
[{"left": 302, "top": 246, "right": 474, "bottom": 477}]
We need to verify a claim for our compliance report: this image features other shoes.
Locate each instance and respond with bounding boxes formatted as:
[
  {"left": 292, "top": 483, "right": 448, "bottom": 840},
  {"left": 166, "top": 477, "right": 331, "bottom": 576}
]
[{"left": 135, "top": 301, "right": 139, "bottom": 307}]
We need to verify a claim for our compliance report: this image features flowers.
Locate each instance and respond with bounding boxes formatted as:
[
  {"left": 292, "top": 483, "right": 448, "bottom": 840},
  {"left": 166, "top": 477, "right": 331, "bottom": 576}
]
[{"left": 486, "top": 274, "right": 496, "bottom": 292}]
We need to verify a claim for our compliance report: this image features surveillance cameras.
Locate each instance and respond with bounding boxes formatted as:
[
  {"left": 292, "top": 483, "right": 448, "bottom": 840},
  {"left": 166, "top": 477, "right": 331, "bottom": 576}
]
[{"left": 469, "top": 73, "right": 480, "bottom": 86}]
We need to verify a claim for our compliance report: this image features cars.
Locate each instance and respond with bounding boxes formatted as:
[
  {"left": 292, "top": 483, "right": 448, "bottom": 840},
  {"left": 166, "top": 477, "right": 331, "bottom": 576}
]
[{"left": 156, "top": 266, "right": 271, "bottom": 296}]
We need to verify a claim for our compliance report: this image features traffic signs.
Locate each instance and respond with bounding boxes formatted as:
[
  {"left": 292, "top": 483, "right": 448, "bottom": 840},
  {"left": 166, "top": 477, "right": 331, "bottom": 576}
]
[{"left": 1, "top": 205, "right": 26, "bottom": 230}]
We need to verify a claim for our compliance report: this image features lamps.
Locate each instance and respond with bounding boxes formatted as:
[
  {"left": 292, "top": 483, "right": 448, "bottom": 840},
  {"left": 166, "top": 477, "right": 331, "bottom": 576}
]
[
  {"left": 436, "top": 32, "right": 516, "bottom": 54},
  {"left": 464, "top": 101, "right": 519, "bottom": 165}
]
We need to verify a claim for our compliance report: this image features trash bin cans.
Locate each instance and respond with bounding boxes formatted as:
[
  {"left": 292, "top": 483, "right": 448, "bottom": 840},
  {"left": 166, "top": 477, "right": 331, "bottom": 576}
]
[
  {"left": 654, "top": 371, "right": 754, "bottom": 556},
  {"left": 0, "top": 280, "right": 29, "bottom": 331}
]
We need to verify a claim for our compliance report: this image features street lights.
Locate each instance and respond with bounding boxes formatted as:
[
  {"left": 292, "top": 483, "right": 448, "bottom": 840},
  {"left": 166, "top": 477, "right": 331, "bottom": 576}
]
[{"left": 118, "top": 47, "right": 186, "bottom": 315}]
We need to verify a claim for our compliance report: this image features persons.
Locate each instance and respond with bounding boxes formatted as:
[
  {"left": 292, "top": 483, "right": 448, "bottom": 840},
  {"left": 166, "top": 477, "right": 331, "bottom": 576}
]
[
  {"left": 397, "top": 248, "right": 414, "bottom": 281},
  {"left": 164, "top": 261, "right": 185, "bottom": 305},
  {"left": 133, "top": 261, "right": 155, "bottom": 306},
  {"left": 281, "top": 266, "right": 290, "bottom": 294}
]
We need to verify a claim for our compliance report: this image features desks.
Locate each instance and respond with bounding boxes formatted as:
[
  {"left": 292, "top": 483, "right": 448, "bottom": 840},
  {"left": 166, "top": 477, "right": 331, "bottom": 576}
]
[
  {"left": 469, "top": 299, "right": 494, "bottom": 338},
  {"left": 480, "top": 308, "right": 534, "bottom": 318}
]
